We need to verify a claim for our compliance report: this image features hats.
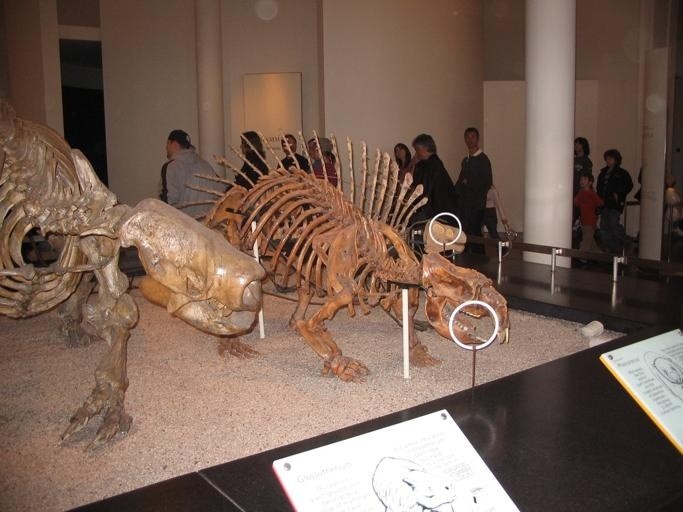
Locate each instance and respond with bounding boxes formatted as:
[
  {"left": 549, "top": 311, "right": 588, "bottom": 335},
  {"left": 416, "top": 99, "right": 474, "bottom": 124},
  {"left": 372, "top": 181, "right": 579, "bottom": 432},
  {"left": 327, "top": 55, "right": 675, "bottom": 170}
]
[
  {"left": 309, "top": 138, "right": 333, "bottom": 149},
  {"left": 168, "top": 130, "right": 196, "bottom": 149}
]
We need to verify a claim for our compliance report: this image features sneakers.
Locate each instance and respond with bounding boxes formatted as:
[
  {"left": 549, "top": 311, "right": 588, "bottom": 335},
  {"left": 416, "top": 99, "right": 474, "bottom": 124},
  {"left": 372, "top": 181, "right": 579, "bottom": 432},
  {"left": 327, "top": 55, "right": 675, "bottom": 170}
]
[{"left": 502, "top": 249, "right": 511, "bottom": 257}]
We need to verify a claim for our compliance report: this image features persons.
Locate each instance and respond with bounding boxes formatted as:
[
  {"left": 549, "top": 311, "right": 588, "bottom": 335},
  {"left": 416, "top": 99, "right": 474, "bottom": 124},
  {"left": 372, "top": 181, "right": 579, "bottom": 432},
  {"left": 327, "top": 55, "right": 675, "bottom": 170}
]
[
  {"left": 572, "top": 136, "right": 642, "bottom": 266},
  {"left": 160, "top": 129, "right": 226, "bottom": 218},
  {"left": 226, "top": 130, "right": 269, "bottom": 195},
  {"left": 276, "top": 135, "right": 338, "bottom": 190},
  {"left": 454, "top": 127, "right": 510, "bottom": 266},
  {"left": 392, "top": 134, "right": 456, "bottom": 237}
]
